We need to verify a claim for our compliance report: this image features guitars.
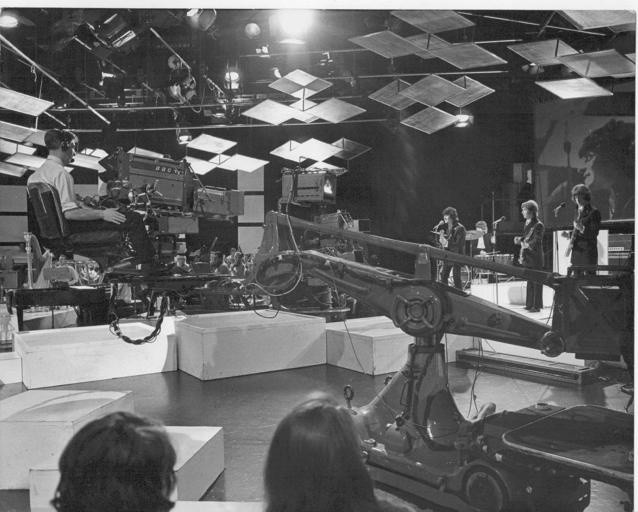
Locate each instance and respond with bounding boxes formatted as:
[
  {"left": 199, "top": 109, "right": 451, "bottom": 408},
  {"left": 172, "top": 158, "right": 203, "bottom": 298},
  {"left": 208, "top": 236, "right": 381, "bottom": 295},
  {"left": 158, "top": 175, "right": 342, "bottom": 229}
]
[
  {"left": 517, "top": 227, "right": 535, "bottom": 265},
  {"left": 564, "top": 206, "right": 589, "bottom": 257},
  {"left": 437, "top": 229, "right": 446, "bottom": 271}
]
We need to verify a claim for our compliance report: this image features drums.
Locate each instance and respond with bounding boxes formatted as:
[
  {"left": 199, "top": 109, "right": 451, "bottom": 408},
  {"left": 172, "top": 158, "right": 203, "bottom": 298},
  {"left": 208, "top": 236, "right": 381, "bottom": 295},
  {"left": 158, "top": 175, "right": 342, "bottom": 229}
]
[
  {"left": 473, "top": 255, "right": 493, "bottom": 275},
  {"left": 439, "top": 264, "right": 471, "bottom": 288},
  {"left": 492, "top": 254, "right": 514, "bottom": 275}
]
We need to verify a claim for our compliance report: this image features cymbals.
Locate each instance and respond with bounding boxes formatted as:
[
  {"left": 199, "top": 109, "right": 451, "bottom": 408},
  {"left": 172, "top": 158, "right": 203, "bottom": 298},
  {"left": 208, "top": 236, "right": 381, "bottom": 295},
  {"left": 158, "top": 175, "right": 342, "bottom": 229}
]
[{"left": 464, "top": 230, "right": 484, "bottom": 241}]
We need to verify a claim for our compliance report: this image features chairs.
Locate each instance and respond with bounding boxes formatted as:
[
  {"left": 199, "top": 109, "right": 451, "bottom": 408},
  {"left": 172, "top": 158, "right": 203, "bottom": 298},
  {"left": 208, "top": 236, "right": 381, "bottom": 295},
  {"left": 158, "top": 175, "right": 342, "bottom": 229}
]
[{"left": 24, "top": 183, "right": 125, "bottom": 266}]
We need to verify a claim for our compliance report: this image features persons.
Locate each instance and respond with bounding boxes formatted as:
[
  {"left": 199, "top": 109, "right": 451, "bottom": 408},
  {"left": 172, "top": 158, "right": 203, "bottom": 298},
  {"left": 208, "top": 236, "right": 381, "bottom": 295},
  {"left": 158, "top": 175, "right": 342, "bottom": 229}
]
[
  {"left": 47, "top": 411, "right": 181, "bottom": 511},
  {"left": 25, "top": 127, "right": 177, "bottom": 277},
  {"left": 432, "top": 207, "right": 467, "bottom": 292},
  {"left": 575, "top": 116, "right": 635, "bottom": 219},
  {"left": 513, "top": 199, "right": 546, "bottom": 313},
  {"left": 49, "top": 246, "right": 260, "bottom": 304},
  {"left": 262, "top": 392, "right": 380, "bottom": 510},
  {"left": 563, "top": 184, "right": 601, "bottom": 276},
  {"left": 470, "top": 220, "right": 501, "bottom": 279}
]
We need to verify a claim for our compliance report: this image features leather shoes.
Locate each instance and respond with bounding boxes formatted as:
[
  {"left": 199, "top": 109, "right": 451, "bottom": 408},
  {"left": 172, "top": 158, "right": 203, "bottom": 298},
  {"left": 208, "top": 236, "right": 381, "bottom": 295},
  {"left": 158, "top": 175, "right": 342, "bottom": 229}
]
[
  {"left": 530, "top": 308, "right": 540, "bottom": 312},
  {"left": 621, "top": 382, "right": 633, "bottom": 395},
  {"left": 159, "top": 261, "right": 177, "bottom": 275}
]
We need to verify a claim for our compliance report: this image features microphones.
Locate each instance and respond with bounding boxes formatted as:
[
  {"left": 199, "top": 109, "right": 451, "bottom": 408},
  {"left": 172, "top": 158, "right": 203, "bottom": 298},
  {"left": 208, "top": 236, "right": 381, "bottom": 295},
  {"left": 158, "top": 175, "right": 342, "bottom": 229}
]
[
  {"left": 554, "top": 201, "right": 566, "bottom": 217},
  {"left": 434, "top": 220, "right": 444, "bottom": 230},
  {"left": 70, "top": 159, "right": 74, "bottom": 162},
  {"left": 493, "top": 215, "right": 506, "bottom": 224}
]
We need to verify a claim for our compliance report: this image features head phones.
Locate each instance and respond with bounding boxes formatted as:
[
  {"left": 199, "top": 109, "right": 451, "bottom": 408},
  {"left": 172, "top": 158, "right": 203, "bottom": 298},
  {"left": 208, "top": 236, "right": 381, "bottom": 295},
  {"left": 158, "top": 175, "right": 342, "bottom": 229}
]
[
  {"left": 61, "top": 130, "right": 68, "bottom": 153},
  {"left": 571, "top": 183, "right": 592, "bottom": 202}
]
